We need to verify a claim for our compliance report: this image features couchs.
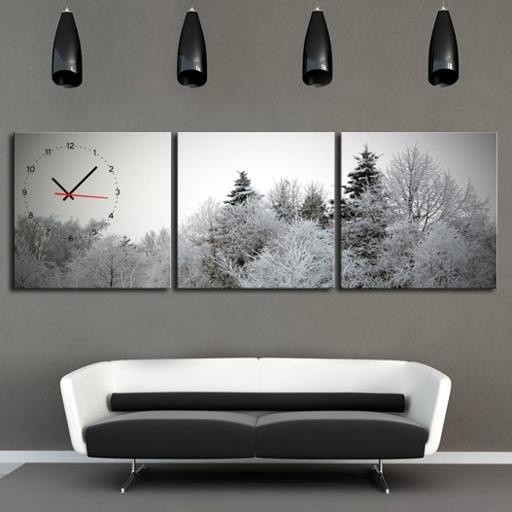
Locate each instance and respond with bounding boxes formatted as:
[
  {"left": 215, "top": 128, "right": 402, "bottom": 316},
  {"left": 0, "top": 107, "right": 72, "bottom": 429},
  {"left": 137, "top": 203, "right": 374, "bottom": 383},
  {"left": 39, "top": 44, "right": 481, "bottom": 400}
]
[{"left": 60, "top": 357, "right": 452, "bottom": 495}]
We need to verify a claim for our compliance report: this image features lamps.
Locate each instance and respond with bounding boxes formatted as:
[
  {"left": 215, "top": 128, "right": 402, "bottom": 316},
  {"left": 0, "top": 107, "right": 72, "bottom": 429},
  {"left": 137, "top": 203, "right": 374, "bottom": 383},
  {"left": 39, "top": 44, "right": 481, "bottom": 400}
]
[
  {"left": 52, "top": 0, "right": 82, "bottom": 88},
  {"left": 302, "top": 0, "right": 332, "bottom": 87},
  {"left": 177, "top": 0, "right": 208, "bottom": 88},
  {"left": 428, "top": 0, "right": 459, "bottom": 86}
]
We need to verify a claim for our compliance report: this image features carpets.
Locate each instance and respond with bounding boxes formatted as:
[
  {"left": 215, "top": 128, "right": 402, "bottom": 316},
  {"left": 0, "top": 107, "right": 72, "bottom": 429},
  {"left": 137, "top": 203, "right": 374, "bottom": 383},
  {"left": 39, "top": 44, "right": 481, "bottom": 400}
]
[{"left": 0, "top": 463, "right": 512, "bottom": 512}]
